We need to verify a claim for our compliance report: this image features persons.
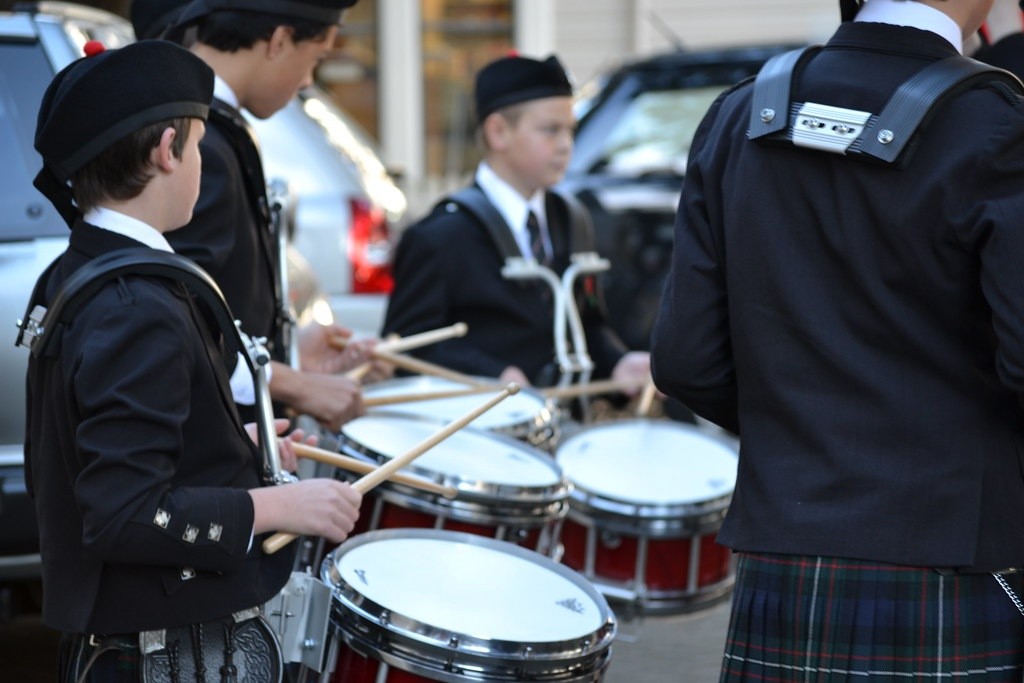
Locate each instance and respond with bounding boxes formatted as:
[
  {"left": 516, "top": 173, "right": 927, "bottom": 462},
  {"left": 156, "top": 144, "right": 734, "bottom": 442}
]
[
  {"left": 646, "top": 0, "right": 1024, "bottom": 683},
  {"left": 144, "top": 0, "right": 403, "bottom": 445},
  {"left": 379, "top": 55, "right": 661, "bottom": 433},
  {"left": 13, "top": 42, "right": 362, "bottom": 683}
]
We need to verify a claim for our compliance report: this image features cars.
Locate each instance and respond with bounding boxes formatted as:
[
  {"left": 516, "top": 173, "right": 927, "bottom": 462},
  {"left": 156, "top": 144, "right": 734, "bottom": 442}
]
[{"left": 549, "top": 40, "right": 818, "bottom": 354}]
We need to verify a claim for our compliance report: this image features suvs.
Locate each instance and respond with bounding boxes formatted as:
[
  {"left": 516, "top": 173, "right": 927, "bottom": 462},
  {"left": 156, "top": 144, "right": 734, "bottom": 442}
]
[{"left": 0, "top": 0, "right": 414, "bottom": 625}]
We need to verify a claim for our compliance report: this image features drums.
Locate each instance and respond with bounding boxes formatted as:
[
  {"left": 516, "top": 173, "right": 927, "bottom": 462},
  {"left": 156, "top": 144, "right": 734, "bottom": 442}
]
[
  {"left": 313, "top": 526, "right": 617, "bottom": 683},
  {"left": 357, "top": 373, "right": 558, "bottom": 459},
  {"left": 552, "top": 419, "right": 740, "bottom": 617},
  {"left": 315, "top": 412, "right": 567, "bottom": 577}
]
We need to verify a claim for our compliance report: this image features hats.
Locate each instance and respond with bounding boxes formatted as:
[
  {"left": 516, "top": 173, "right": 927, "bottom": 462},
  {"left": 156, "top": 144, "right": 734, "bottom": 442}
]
[
  {"left": 474, "top": 54, "right": 573, "bottom": 126},
  {"left": 32, "top": 40, "right": 215, "bottom": 181},
  {"left": 165, "top": 0, "right": 357, "bottom": 29}
]
